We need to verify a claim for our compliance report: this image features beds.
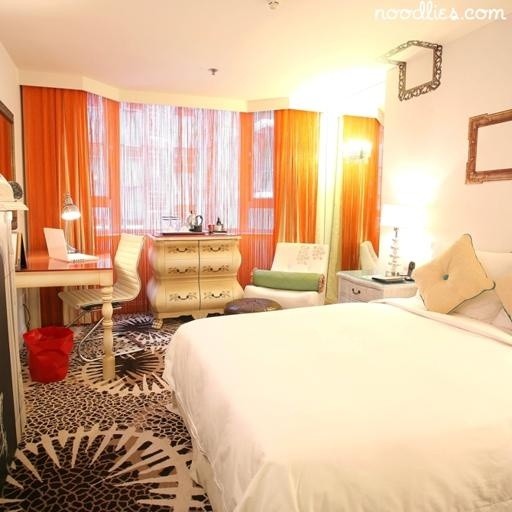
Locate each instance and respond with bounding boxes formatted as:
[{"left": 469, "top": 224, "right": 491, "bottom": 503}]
[{"left": 162, "top": 248, "right": 512, "bottom": 512}]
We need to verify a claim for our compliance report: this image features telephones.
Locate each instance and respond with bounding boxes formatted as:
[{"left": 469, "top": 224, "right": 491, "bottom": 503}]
[{"left": 400, "top": 261, "right": 415, "bottom": 281}]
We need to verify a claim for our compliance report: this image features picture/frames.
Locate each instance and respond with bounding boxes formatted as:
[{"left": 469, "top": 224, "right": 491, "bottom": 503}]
[
  {"left": 0, "top": 100, "right": 18, "bottom": 231},
  {"left": 464, "top": 108, "right": 512, "bottom": 183}
]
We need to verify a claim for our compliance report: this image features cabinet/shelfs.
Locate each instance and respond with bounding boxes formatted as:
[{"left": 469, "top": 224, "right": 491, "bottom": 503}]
[{"left": 145, "top": 232, "right": 245, "bottom": 330}]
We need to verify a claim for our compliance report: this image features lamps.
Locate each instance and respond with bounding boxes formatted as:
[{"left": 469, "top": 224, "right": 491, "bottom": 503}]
[
  {"left": 59, "top": 191, "right": 83, "bottom": 253},
  {"left": 370, "top": 205, "right": 415, "bottom": 284},
  {"left": 339, "top": 139, "right": 373, "bottom": 270}
]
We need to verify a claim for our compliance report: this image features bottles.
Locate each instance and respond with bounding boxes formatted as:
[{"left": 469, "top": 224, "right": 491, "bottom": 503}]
[
  {"left": 215, "top": 217, "right": 223, "bottom": 231},
  {"left": 186, "top": 210, "right": 197, "bottom": 231}
]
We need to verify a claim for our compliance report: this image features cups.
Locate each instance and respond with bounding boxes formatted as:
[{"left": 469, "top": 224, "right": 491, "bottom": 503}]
[
  {"left": 208, "top": 225, "right": 221, "bottom": 233},
  {"left": 160, "top": 216, "right": 180, "bottom": 232}
]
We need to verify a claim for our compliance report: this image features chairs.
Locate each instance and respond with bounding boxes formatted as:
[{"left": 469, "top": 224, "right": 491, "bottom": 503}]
[
  {"left": 244, "top": 242, "right": 330, "bottom": 308},
  {"left": 56, "top": 231, "right": 145, "bottom": 363}
]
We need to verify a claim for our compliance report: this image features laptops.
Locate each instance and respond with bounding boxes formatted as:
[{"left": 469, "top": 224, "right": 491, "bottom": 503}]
[{"left": 43, "top": 227, "right": 99, "bottom": 263}]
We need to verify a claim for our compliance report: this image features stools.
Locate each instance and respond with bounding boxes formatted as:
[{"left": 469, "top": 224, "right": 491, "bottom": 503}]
[{"left": 224, "top": 298, "right": 281, "bottom": 314}]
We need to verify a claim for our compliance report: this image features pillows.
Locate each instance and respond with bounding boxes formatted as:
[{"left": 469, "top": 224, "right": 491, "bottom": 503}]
[
  {"left": 249, "top": 266, "right": 325, "bottom": 294},
  {"left": 493, "top": 266, "right": 512, "bottom": 322},
  {"left": 409, "top": 233, "right": 497, "bottom": 314}
]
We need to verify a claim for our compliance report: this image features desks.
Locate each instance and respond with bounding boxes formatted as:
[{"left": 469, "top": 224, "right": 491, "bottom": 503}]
[{"left": 15, "top": 251, "right": 115, "bottom": 381}]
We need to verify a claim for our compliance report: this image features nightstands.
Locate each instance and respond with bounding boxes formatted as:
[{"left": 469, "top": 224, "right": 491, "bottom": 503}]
[{"left": 334, "top": 269, "right": 417, "bottom": 304}]
